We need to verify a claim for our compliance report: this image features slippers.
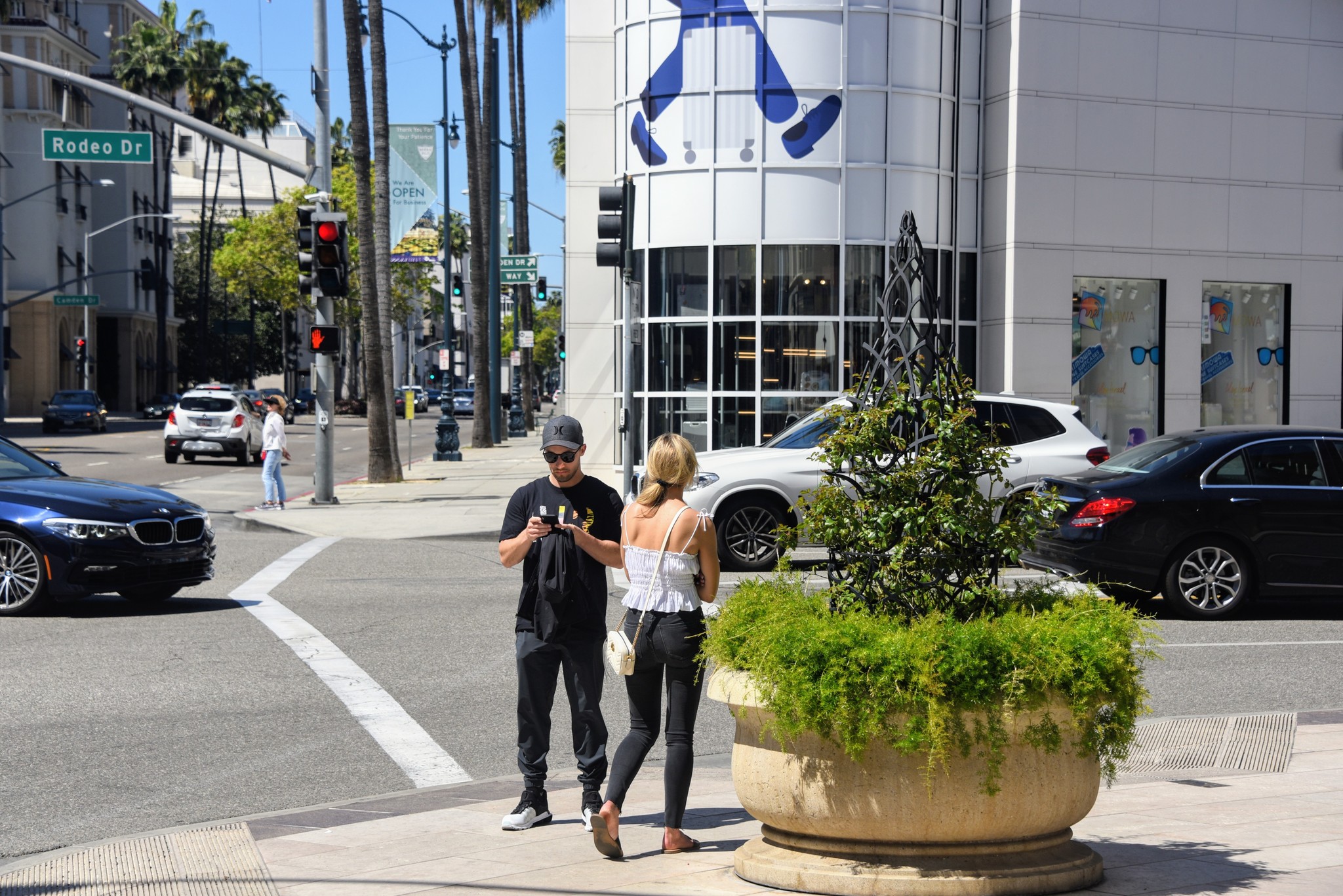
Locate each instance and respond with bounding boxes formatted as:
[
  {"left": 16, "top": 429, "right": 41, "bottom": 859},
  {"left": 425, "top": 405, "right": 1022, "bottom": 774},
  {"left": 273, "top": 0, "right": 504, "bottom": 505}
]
[
  {"left": 662, "top": 837, "right": 702, "bottom": 854},
  {"left": 591, "top": 815, "right": 625, "bottom": 858}
]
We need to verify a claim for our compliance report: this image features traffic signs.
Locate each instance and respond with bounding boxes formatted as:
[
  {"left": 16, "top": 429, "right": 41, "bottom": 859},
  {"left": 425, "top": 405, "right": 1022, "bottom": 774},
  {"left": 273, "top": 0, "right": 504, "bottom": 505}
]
[
  {"left": 468, "top": 255, "right": 538, "bottom": 270},
  {"left": 468, "top": 270, "right": 538, "bottom": 284}
]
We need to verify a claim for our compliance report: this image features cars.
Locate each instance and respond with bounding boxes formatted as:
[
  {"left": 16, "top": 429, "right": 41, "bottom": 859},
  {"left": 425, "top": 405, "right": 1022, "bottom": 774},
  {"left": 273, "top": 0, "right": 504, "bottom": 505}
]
[
  {"left": 532, "top": 386, "right": 542, "bottom": 412},
  {"left": 551, "top": 389, "right": 561, "bottom": 405},
  {"left": 452, "top": 388, "right": 475, "bottom": 416},
  {"left": 1018, "top": 425, "right": 1343, "bottom": 624},
  {"left": 395, "top": 385, "right": 443, "bottom": 413},
  {"left": 41, "top": 389, "right": 109, "bottom": 434},
  {"left": 0, "top": 436, "right": 217, "bottom": 615},
  {"left": 140, "top": 382, "right": 316, "bottom": 466}
]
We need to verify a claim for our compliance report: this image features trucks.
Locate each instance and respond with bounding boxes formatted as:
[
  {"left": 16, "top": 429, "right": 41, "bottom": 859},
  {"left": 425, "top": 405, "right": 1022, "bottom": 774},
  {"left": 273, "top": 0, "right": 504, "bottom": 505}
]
[{"left": 500, "top": 357, "right": 512, "bottom": 410}]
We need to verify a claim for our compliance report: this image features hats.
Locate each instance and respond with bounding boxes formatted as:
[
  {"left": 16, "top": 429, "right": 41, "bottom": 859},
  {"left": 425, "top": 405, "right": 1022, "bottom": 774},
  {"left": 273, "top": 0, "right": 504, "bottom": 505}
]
[
  {"left": 540, "top": 414, "right": 584, "bottom": 450},
  {"left": 264, "top": 396, "right": 281, "bottom": 406}
]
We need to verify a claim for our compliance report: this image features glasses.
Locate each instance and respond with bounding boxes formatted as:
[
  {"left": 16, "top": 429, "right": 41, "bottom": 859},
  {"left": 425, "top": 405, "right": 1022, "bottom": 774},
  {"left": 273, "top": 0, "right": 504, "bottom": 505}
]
[
  {"left": 268, "top": 401, "right": 277, "bottom": 406},
  {"left": 543, "top": 445, "right": 582, "bottom": 463}
]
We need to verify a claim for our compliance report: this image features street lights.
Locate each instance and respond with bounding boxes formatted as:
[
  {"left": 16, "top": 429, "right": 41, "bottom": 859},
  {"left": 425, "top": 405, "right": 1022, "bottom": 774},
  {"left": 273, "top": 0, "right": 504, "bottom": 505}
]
[
  {"left": 462, "top": 189, "right": 565, "bottom": 415},
  {"left": 449, "top": 112, "right": 527, "bottom": 437},
  {"left": 0, "top": 178, "right": 116, "bottom": 441},
  {"left": 352, "top": 1, "right": 462, "bottom": 462},
  {"left": 83, "top": 213, "right": 182, "bottom": 390}
]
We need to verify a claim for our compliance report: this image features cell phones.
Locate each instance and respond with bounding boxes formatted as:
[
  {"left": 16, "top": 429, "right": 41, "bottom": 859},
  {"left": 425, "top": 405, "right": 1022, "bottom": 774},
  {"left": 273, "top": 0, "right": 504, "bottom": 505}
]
[{"left": 540, "top": 514, "right": 561, "bottom": 535}]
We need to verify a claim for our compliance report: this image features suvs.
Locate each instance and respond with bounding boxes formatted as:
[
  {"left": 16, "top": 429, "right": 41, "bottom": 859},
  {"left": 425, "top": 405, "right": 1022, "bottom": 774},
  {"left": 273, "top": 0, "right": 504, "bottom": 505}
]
[{"left": 622, "top": 391, "right": 1111, "bottom": 573}]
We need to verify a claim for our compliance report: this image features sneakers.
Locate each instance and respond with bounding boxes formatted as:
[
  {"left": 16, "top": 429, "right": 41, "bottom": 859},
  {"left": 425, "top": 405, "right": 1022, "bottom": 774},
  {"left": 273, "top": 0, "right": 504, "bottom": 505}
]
[
  {"left": 502, "top": 789, "right": 552, "bottom": 830},
  {"left": 254, "top": 501, "right": 276, "bottom": 511},
  {"left": 580, "top": 790, "right": 605, "bottom": 831},
  {"left": 274, "top": 502, "right": 285, "bottom": 510}
]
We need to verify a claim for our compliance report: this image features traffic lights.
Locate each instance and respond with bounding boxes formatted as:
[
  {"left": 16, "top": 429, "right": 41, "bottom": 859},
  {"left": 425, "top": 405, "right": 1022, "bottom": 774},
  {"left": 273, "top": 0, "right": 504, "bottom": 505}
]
[
  {"left": 597, "top": 173, "right": 629, "bottom": 267},
  {"left": 309, "top": 325, "right": 341, "bottom": 353},
  {"left": 536, "top": 277, "right": 547, "bottom": 301},
  {"left": 295, "top": 204, "right": 350, "bottom": 298},
  {"left": 452, "top": 272, "right": 464, "bottom": 297},
  {"left": 557, "top": 332, "right": 566, "bottom": 361},
  {"left": 73, "top": 337, "right": 86, "bottom": 374}
]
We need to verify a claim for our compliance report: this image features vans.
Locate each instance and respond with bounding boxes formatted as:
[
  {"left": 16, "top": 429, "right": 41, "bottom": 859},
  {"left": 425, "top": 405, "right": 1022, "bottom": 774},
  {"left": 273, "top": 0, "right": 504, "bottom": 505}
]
[{"left": 468, "top": 374, "right": 475, "bottom": 389}]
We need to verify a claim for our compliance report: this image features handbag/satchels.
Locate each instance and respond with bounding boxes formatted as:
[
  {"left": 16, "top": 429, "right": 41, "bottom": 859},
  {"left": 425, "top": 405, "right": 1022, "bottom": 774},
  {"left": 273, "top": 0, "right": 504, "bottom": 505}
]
[
  {"left": 606, "top": 629, "right": 640, "bottom": 675},
  {"left": 261, "top": 451, "right": 267, "bottom": 460}
]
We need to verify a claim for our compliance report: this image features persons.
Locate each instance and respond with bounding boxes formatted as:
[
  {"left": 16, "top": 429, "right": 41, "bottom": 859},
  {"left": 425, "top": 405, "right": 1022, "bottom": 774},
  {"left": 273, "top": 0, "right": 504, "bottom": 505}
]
[
  {"left": 499, "top": 415, "right": 624, "bottom": 831},
  {"left": 590, "top": 432, "right": 720, "bottom": 858},
  {"left": 254, "top": 394, "right": 291, "bottom": 511}
]
